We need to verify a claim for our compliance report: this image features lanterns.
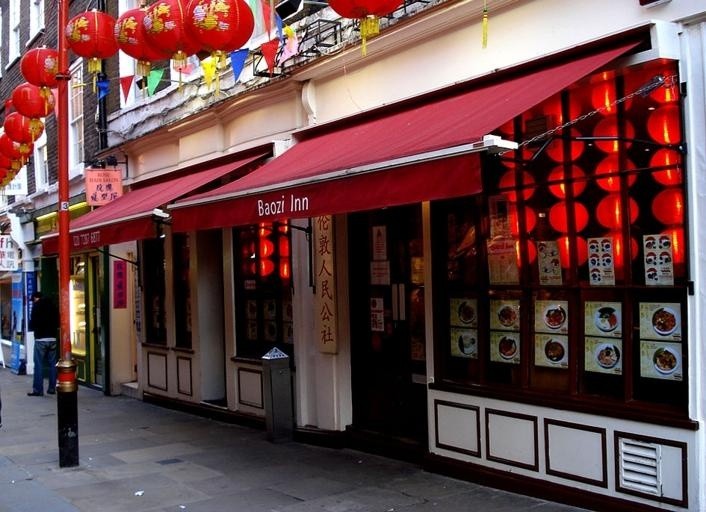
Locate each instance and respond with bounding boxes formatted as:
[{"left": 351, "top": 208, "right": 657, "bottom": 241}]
[
  {"left": 245, "top": 218, "right": 291, "bottom": 279},
  {"left": 65, "top": 0, "right": 255, "bottom": 81},
  {"left": 0, "top": 44, "right": 58, "bottom": 190},
  {"left": 327, "top": 0, "right": 403, "bottom": 39},
  {"left": 496, "top": 65, "right": 685, "bottom": 274}
]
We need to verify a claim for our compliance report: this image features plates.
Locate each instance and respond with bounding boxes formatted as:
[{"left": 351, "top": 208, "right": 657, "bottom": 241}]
[
  {"left": 456, "top": 300, "right": 477, "bottom": 323},
  {"left": 457, "top": 333, "right": 477, "bottom": 355},
  {"left": 650, "top": 307, "right": 678, "bottom": 335},
  {"left": 494, "top": 305, "right": 519, "bottom": 328},
  {"left": 543, "top": 305, "right": 566, "bottom": 328},
  {"left": 497, "top": 336, "right": 518, "bottom": 359},
  {"left": 594, "top": 344, "right": 621, "bottom": 368},
  {"left": 594, "top": 306, "right": 619, "bottom": 331},
  {"left": 542, "top": 338, "right": 567, "bottom": 363},
  {"left": 652, "top": 346, "right": 679, "bottom": 373}
]
